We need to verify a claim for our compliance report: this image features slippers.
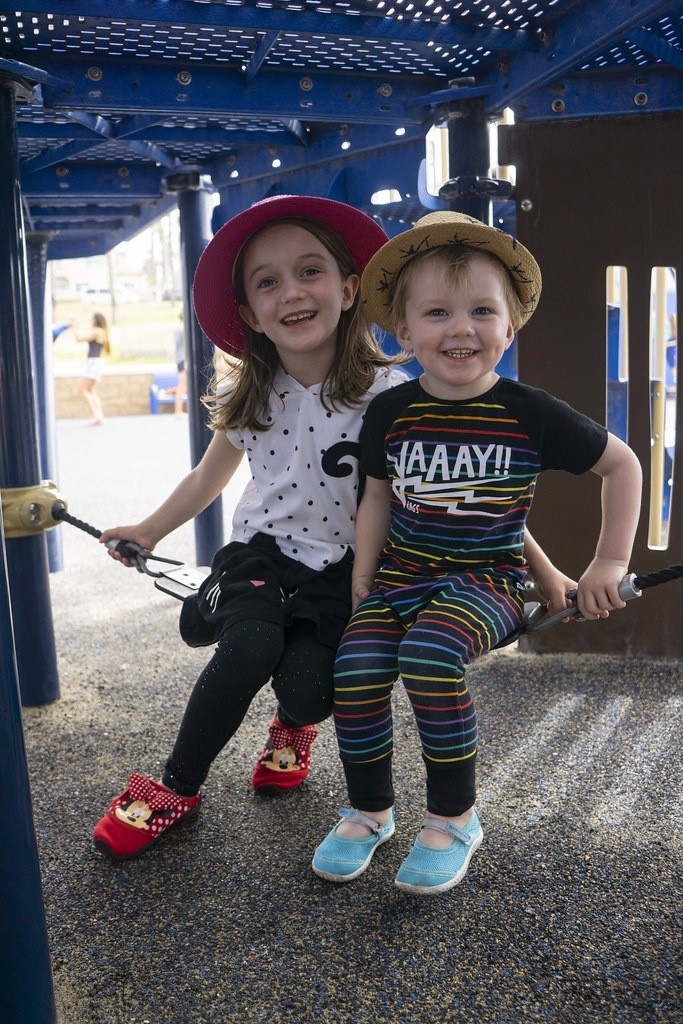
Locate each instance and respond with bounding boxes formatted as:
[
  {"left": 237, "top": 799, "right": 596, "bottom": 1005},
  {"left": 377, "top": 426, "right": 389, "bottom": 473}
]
[
  {"left": 252, "top": 711, "right": 319, "bottom": 795},
  {"left": 93, "top": 773, "right": 202, "bottom": 863}
]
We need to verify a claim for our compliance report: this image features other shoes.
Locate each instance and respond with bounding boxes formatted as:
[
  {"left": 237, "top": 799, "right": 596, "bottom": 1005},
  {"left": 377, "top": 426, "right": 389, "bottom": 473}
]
[
  {"left": 312, "top": 803, "right": 396, "bottom": 882},
  {"left": 394, "top": 809, "right": 483, "bottom": 895}
]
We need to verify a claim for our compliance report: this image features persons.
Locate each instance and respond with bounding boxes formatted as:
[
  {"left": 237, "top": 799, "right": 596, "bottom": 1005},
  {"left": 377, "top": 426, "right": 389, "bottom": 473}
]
[
  {"left": 312, "top": 211, "right": 642, "bottom": 895},
  {"left": 70, "top": 313, "right": 111, "bottom": 424},
  {"left": 175, "top": 311, "right": 189, "bottom": 415},
  {"left": 93, "top": 195, "right": 585, "bottom": 863}
]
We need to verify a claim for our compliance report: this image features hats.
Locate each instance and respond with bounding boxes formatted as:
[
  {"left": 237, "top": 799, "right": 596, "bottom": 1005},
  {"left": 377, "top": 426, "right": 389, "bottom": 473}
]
[
  {"left": 192, "top": 195, "right": 390, "bottom": 361},
  {"left": 361, "top": 210, "right": 542, "bottom": 335}
]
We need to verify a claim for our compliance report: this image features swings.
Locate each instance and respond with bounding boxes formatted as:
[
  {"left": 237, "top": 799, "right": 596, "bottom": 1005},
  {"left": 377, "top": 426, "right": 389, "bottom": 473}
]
[{"left": 147, "top": 213, "right": 184, "bottom": 303}]
